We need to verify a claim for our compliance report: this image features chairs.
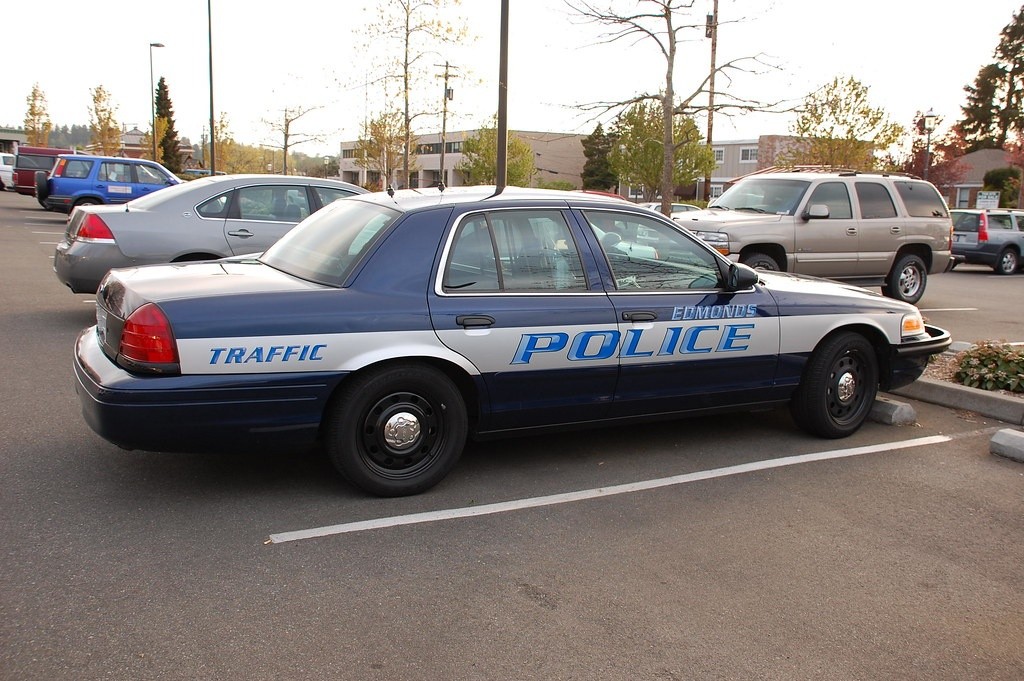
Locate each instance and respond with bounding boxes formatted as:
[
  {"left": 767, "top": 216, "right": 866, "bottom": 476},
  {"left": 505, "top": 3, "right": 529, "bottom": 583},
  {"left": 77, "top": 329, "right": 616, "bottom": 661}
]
[{"left": 275, "top": 198, "right": 300, "bottom": 220}]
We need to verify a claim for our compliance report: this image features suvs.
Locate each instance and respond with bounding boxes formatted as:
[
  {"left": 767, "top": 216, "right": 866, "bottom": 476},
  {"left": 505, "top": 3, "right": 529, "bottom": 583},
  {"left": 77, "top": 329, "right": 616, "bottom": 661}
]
[
  {"left": 634, "top": 167, "right": 955, "bottom": 305},
  {"left": 949, "top": 208, "right": 1024, "bottom": 276},
  {"left": 34, "top": 153, "right": 189, "bottom": 215}
]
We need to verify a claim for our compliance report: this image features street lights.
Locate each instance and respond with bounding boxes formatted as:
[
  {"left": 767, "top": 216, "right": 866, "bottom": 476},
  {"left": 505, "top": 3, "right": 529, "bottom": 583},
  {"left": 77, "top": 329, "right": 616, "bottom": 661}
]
[
  {"left": 149, "top": 42, "right": 167, "bottom": 161},
  {"left": 324, "top": 155, "right": 330, "bottom": 178},
  {"left": 695, "top": 178, "right": 700, "bottom": 202},
  {"left": 921, "top": 108, "right": 938, "bottom": 180},
  {"left": 120, "top": 139, "right": 126, "bottom": 157}
]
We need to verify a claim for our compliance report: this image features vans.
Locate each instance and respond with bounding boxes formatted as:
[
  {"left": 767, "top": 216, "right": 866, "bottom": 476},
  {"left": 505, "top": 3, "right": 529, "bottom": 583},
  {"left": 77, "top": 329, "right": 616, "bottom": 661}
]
[
  {"left": 11, "top": 146, "right": 89, "bottom": 198},
  {"left": 0, "top": 152, "right": 41, "bottom": 191}
]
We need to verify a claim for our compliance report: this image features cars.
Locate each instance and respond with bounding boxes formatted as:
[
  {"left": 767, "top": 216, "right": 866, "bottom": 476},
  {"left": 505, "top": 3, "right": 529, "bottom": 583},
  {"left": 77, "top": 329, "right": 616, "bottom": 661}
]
[
  {"left": 182, "top": 168, "right": 228, "bottom": 176},
  {"left": 69, "top": 170, "right": 954, "bottom": 499},
  {"left": 615, "top": 202, "right": 703, "bottom": 237},
  {"left": 50, "top": 174, "right": 390, "bottom": 296}
]
